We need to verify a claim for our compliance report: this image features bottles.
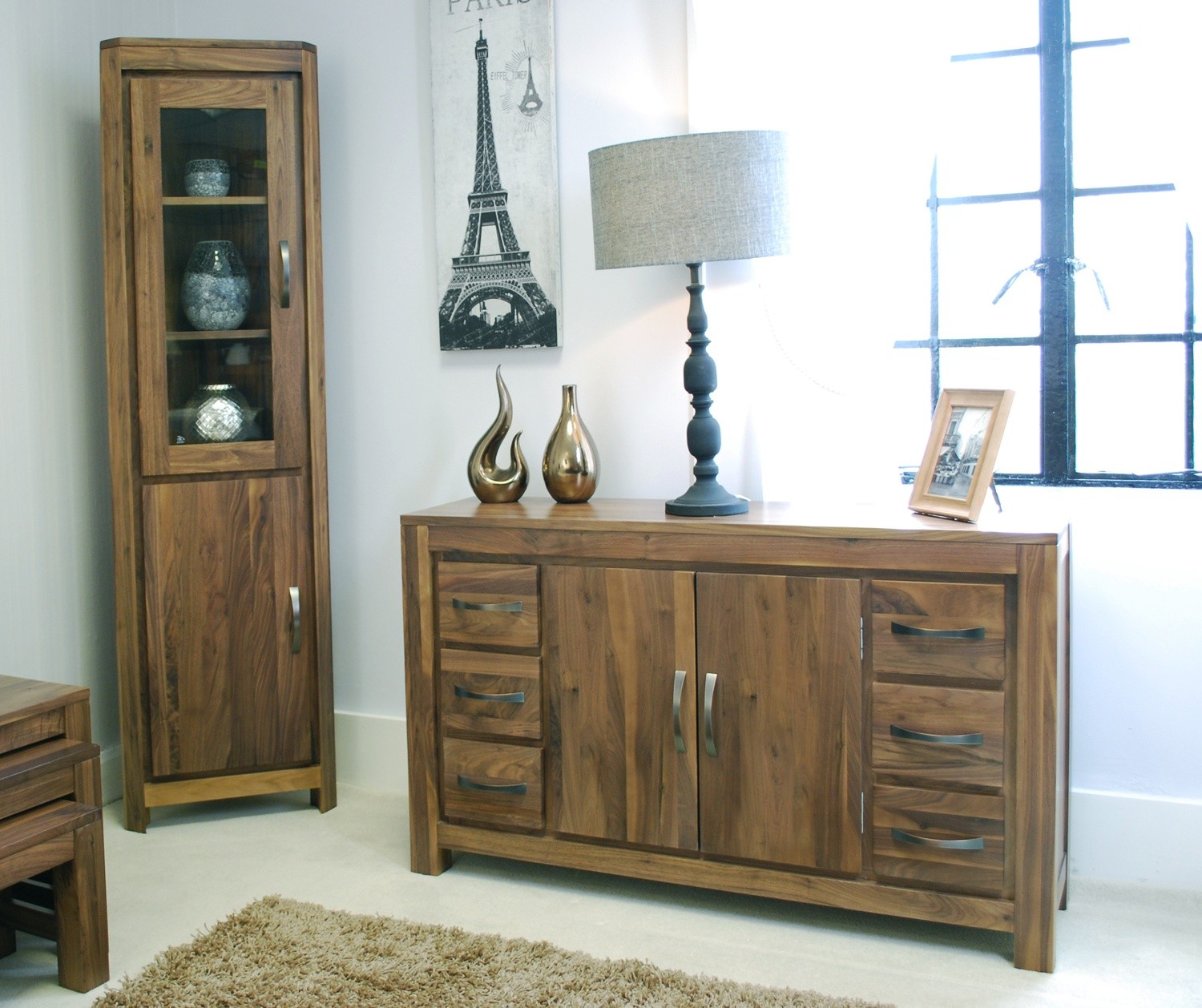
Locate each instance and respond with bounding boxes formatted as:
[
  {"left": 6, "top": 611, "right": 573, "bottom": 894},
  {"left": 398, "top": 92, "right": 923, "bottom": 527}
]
[
  {"left": 185, "top": 385, "right": 253, "bottom": 445},
  {"left": 181, "top": 240, "right": 252, "bottom": 332},
  {"left": 542, "top": 384, "right": 600, "bottom": 504}
]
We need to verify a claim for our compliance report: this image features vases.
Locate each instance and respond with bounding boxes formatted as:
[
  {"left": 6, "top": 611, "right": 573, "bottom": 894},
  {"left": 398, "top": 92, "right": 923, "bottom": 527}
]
[
  {"left": 541, "top": 384, "right": 601, "bottom": 505},
  {"left": 181, "top": 241, "right": 252, "bottom": 330},
  {"left": 185, "top": 384, "right": 252, "bottom": 443}
]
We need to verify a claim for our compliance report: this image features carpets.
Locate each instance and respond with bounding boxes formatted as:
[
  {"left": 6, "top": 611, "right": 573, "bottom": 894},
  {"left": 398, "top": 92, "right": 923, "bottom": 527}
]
[{"left": 90, "top": 896, "right": 898, "bottom": 1008}]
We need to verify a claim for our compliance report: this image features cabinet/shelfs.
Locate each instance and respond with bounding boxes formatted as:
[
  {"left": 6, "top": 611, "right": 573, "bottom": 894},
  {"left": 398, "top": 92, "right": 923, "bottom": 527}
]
[
  {"left": 98, "top": 38, "right": 337, "bottom": 832},
  {"left": 400, "top": 494, "right": 1069, "bottom": 974}
]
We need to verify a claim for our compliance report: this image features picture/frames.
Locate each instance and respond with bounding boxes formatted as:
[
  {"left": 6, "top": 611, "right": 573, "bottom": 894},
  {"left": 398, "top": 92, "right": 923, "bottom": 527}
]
[{"left": 909, "top": 388, "right": 1016, "bottom": 524}]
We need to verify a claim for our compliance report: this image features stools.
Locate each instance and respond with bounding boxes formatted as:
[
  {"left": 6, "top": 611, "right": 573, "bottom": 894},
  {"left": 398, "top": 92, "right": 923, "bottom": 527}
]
[{"left": 0, "top": 674, "right": 112, "bottom": 993}]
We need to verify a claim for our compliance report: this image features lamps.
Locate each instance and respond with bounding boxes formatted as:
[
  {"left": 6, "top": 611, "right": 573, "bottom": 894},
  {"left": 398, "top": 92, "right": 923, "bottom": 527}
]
[{"left": 588, "top": 130, "right": 792, "bottom": 516}]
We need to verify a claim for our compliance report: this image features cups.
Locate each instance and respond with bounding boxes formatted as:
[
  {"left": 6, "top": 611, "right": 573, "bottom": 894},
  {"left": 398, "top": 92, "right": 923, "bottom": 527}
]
[{"left": 184, "top": 159, "right": 231, "bottom": 197}]
